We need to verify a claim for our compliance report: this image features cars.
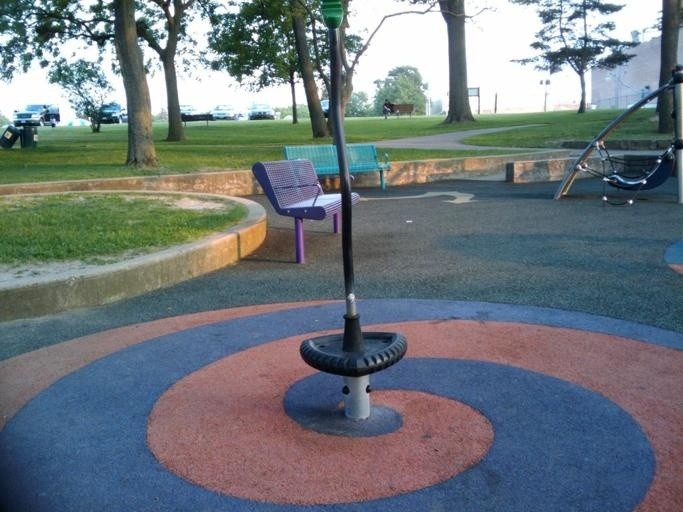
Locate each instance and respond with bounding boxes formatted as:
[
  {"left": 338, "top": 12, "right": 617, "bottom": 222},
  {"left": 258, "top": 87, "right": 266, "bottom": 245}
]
[
  {"left": 95, "top": 102, "right": 127, "bottom": 124},
  {"left": 319, "top": 99, "right": 331, "bottom": 119},
  {"left": 247, "top": 103, "right": 276, "bottom": 120},
  {"left": 209, "top": 103, "right": 240, "bottom": 121},
  {"left": 179, "top": 104, "right": 210, "bottom": 121}
]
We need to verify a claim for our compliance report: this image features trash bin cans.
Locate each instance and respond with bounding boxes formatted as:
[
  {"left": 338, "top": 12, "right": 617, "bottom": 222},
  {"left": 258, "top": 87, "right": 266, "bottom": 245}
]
[{"left": 0, "top": 120, "right": 42, "bottom": 149}]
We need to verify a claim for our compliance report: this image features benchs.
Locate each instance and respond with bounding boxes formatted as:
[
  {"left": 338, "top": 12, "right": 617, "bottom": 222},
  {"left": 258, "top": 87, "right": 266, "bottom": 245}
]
[
  {"left": 252, "top": 159, "right": 360, "bottom": 264},
  {"left": 384, "top": 104, "right": 414, "bottom": 120},
  {"left": 182, "top": 114, "right": 216, "bottom": 127},
  {"left": 282, "top": 143, "right": 391, "bottom": 194}
]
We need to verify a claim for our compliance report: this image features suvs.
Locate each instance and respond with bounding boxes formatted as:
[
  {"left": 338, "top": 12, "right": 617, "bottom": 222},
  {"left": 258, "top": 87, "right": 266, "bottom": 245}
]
[{"left": 14, "top": 103, "right": 59, "bottom": 126}]
